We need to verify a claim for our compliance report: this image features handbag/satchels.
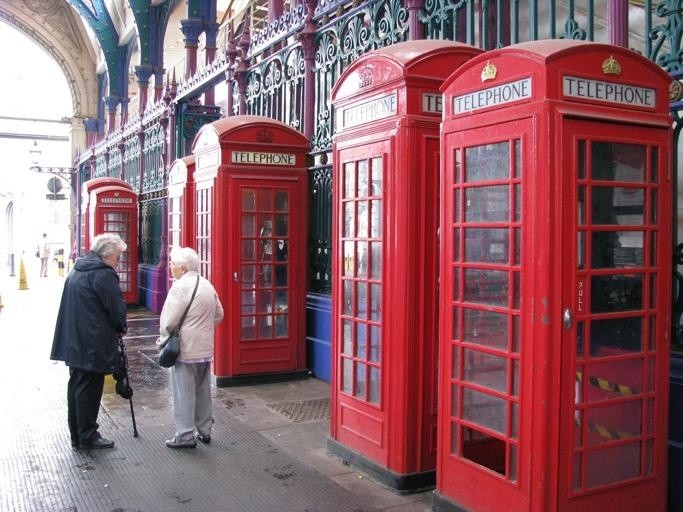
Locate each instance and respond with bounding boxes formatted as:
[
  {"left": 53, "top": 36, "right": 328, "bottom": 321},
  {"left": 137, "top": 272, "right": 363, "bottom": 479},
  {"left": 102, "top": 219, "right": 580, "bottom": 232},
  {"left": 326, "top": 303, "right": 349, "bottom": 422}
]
[{"left": 157, "top": 331, "right": 181, "bottom": 369}]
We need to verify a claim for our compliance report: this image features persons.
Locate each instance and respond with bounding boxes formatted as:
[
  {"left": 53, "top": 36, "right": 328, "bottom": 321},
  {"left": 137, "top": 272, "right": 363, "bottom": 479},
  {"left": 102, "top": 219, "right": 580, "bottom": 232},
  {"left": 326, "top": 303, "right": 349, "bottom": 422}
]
[
  {"left": 37, "top": 233, "right": 50, "bottom": 277},
  {"left": 155, "top": 247, "right": 225, "bottom": 447},
  {"left": 50, "top": 233, "right": 130, "bottom": 449}
]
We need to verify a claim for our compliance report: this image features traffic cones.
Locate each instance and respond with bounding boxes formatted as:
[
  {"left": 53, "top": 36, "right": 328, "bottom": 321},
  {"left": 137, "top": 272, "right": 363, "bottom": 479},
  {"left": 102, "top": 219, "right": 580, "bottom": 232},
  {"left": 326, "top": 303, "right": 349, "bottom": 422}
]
[{"left": 16, "top": 259, "right": 30, "bottom": 291}]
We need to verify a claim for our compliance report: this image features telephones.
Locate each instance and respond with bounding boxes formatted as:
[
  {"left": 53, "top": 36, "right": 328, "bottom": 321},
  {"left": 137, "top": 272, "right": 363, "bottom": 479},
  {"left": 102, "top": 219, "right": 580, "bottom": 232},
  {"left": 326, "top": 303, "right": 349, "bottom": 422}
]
[{"left": 260, "top": 220, "right": 272, "bottom": 255}]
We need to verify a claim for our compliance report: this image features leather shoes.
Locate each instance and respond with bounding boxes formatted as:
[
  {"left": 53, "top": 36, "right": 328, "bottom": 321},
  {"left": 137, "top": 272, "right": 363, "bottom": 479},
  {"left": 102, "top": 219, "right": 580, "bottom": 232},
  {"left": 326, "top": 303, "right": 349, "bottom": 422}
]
[
  {"left": 79, "top": 438, "right": 115, "bottom": 450},
  {"left": 165, "top": 433, "right": 197, "bottom": 449},
  {"left": 195, "top": 432, "right": 211, "bottom": 444}
]
